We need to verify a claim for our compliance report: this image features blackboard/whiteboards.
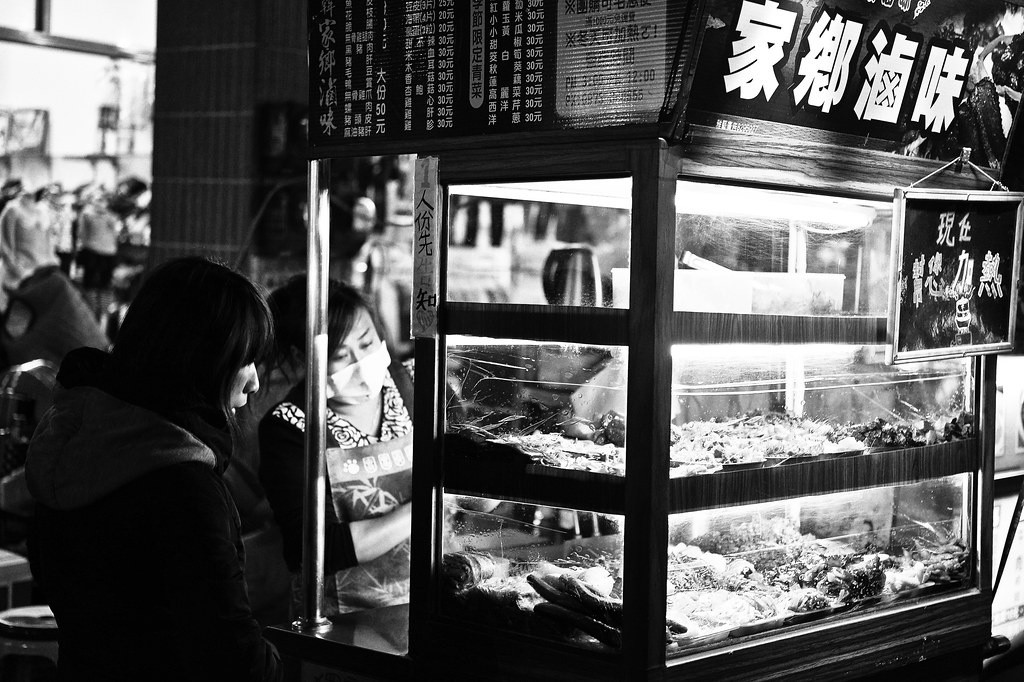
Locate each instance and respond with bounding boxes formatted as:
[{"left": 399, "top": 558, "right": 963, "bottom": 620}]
[{"left": 883, "top": 185, "right": 1024, "bottom": 365}]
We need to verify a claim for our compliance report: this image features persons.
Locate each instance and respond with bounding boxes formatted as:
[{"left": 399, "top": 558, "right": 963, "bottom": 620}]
[
  {"left": 258, "top": 272, "right": 411, "bottom": 618},
  {"left": 20, "top": 254, "right": 282, "bottom": 682},
  {"left": 0, "top": 174, "right": 150, "bottom": 452}
]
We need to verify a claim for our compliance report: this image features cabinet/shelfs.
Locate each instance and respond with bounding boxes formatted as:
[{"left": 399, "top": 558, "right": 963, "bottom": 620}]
[{"left": 405, "top": 124, "right": 1003, "bottom": 682}]
